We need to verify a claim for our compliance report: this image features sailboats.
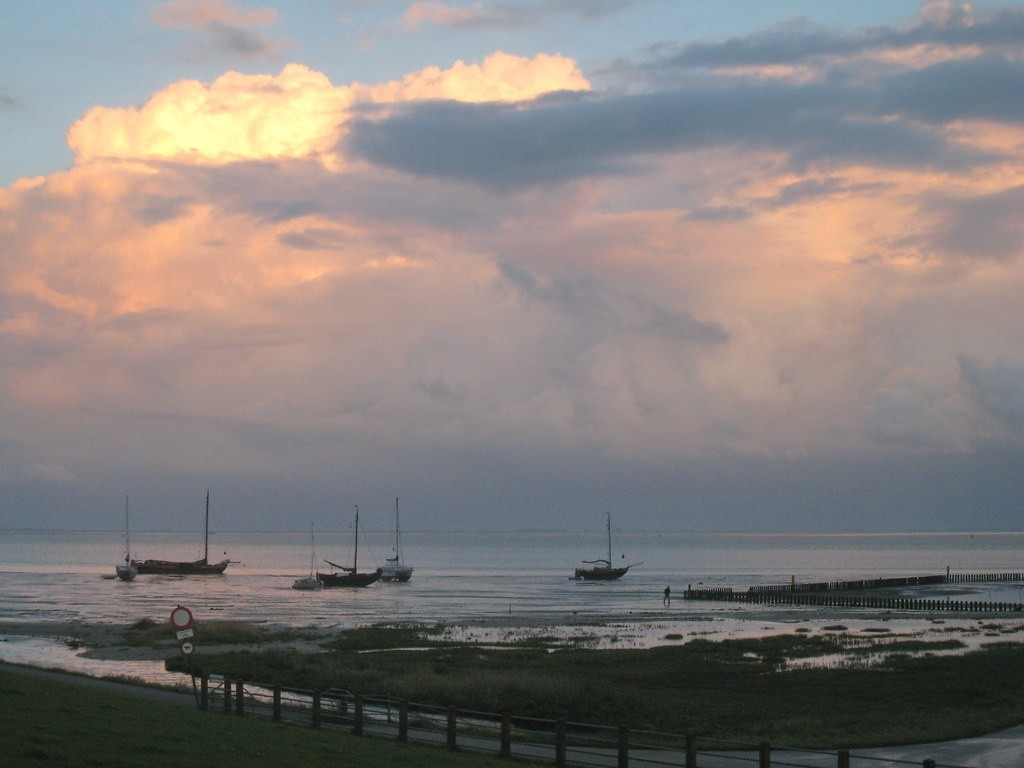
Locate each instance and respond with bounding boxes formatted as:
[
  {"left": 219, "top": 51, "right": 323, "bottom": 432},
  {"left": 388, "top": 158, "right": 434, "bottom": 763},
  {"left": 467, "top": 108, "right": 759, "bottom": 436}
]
[
  {"left": 292, "top": 495, "right": 413, "bottom": 589},
  {"left": 115, "top": 487, "right": 231, "bottom": 580},
  {"left": 575, "top": 512, "right": 629, "bottom": 579}
]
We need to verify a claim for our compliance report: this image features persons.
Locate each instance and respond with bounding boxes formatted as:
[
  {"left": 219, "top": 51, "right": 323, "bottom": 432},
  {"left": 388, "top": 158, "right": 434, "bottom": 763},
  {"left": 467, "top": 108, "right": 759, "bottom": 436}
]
[{"left": 663, "top": 586, "right": 670, "bottom": 605}]
